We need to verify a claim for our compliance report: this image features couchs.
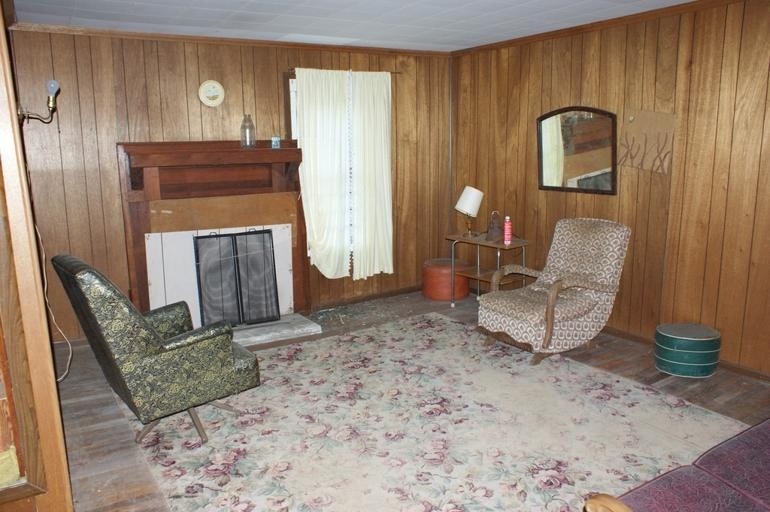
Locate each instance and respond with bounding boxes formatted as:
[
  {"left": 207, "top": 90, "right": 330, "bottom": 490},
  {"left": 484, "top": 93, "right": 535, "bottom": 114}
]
[{"left": 615, "top": 415, "right": 770, "bottom": 512}]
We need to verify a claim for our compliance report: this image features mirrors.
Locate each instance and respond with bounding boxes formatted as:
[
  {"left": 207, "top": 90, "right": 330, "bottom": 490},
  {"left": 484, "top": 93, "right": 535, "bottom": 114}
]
[{"left": 536, "top": 105, "right": 618, "bottom": 196}]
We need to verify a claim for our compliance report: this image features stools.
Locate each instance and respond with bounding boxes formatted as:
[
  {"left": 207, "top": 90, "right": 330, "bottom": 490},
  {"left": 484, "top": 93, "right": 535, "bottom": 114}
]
[
  {"left": 652, "top": 322, "right": 722, "bottom": 379},
  {"left": 422, "top": 258, "right": 470, "bottom": 302}
]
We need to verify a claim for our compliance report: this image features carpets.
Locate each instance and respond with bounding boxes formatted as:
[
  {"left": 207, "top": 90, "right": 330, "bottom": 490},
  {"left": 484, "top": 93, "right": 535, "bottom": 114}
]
[{"left": 109, "top": 310, "right": 749, "bottom": 511}]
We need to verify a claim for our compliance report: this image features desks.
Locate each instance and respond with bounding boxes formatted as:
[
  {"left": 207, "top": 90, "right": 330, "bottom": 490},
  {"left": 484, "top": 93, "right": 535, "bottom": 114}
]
[{"left": 445, "top": 231, "right": 529, "bottom": 309}]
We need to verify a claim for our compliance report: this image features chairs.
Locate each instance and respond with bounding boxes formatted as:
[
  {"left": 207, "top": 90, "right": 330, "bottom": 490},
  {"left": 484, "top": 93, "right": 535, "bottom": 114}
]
[
  {"left": 471, "top": 216, "right": 633, "bottom": 365},
  {"left": 50, "top": 252, "right": 261, "bottom": 445}
]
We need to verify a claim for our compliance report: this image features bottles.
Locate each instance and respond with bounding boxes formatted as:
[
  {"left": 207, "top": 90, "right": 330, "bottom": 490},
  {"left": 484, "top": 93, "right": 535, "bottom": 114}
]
[
  {"left": 504, "top": 215, "right": 512, "bottom": 246},
  {"left": 240, "top": 115, "right": 256, "bottom": 150}
]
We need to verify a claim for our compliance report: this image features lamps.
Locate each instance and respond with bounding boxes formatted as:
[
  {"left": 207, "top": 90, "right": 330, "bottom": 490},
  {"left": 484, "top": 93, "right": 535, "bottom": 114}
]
[
  {"left": 17, "top": 79, "right": 59, "bottom": 120},
  {"left": 454, "top": 186, "right": 484, "bottom": 240}
]
